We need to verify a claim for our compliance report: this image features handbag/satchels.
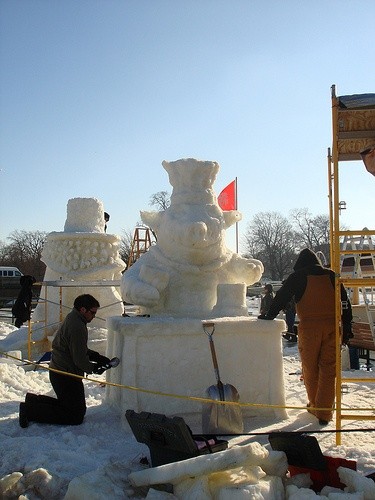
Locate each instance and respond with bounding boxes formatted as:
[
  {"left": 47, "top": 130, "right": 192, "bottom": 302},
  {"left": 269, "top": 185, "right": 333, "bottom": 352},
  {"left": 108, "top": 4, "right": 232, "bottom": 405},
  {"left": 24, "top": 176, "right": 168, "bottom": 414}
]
[{"left": 268, "top": 431, "right": 327, "bottom": 469}]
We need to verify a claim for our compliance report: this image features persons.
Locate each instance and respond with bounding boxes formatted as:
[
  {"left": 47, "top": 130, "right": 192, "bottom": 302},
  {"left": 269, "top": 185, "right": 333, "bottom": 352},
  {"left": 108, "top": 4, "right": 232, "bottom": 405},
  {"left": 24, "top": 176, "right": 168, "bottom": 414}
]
[
  {"left": 282, "top": 280, "right": 298, "bottom": 343},
  {"left": 261, "top": 284, "right": 273, "bottom": 317},
  {"left": 18, "top": 294, "right": 113, "bottom": 428},
  {"left": 259, "top": 248, "right": 355, "bottom": 426}
]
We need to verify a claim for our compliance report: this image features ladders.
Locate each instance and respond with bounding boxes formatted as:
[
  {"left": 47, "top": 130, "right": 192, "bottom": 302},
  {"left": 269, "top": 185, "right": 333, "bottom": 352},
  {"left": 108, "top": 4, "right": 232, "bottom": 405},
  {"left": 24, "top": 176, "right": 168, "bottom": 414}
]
[
  {"left": 127, "top": 227, "right": 152, "bottom": 269},
  {"left": 338, "top": 228, "right": 375, "bottom": 339}
]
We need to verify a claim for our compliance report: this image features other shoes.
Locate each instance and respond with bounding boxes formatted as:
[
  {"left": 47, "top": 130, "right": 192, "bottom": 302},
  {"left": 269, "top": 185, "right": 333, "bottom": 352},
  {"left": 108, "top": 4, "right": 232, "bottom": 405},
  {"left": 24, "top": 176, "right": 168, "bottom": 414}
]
[
  {"left": 282, "top": 334, "right": 289, "bottom": 340},
  {"left": 25, "top": 393, "right": 37, "bottom": 403},
  {"left": 288, "top": 337, "right": 297, "bottom": 342},
  {"left": 306, "top": 403, "right": 328, "bottom": 426},
  {"left": 19, "top": 402, "right": 29, "bottom": 428}
]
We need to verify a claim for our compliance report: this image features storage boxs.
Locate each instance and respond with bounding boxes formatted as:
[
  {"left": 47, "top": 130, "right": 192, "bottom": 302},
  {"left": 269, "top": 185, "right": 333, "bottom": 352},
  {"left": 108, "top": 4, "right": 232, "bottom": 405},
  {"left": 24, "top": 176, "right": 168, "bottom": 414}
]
[
  {"left": 125, "top": 409, "right": 229, "bottom": 467},
  {"left": 268, "top": 432, "right": 356, "bottom": 492}
]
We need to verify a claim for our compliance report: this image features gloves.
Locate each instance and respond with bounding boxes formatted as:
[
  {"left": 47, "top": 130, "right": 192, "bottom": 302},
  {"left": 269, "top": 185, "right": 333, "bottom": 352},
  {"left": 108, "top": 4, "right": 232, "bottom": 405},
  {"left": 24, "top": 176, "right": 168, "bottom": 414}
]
[{"left": 93, "top": 355, "right": 111, "bottom": 375}]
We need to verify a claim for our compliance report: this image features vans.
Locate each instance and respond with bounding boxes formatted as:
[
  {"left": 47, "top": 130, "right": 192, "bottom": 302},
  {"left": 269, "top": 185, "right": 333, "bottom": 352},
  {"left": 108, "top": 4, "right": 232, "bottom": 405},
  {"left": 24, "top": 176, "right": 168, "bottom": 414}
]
[{"left": 0, "top": 267, "right": 24, "bottom": 277}]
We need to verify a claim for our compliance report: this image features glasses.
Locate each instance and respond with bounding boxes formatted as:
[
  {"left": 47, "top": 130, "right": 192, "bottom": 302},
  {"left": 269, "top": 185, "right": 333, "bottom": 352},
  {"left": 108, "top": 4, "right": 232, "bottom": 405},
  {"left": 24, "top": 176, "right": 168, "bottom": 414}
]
[{"left": 84, "top": 307, "right": 97, "bottom": 315}]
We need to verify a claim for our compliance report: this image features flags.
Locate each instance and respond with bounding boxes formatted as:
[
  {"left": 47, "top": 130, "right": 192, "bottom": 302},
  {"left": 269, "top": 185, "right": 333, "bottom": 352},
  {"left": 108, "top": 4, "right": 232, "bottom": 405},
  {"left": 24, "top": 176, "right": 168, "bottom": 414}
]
[{"left": 217, "top": 180, "right": 236, "bottom": 211}]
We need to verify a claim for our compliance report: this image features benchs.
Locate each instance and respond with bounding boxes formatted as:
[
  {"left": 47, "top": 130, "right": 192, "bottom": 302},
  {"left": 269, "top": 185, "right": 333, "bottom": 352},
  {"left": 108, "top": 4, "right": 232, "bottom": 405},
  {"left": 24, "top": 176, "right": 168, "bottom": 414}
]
[{"left": 349, "top": 322, "right": 375, "bottom": 371}]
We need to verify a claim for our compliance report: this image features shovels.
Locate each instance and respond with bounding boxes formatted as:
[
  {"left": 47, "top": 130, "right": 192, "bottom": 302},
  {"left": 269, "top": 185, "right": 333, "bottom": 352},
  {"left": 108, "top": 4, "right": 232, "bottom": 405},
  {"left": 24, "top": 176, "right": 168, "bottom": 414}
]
[{"left": 202, "top": 321, "right": 244, "bottom": 436}]
